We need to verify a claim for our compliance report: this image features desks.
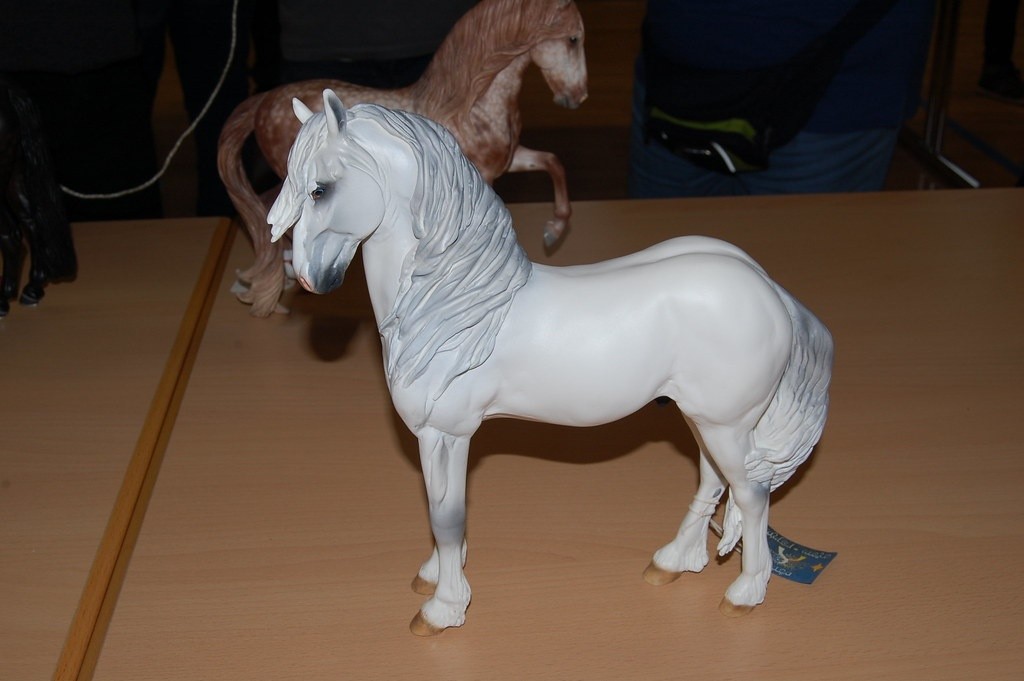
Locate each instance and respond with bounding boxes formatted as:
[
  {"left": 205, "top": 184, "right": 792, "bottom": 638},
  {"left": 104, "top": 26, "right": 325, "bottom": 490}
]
[
  {"left": 77, "top": 187, "right": 1024, "bottom": 681},
  {"left": 0, "top": 216, "right": 230, "bottom": 681}
]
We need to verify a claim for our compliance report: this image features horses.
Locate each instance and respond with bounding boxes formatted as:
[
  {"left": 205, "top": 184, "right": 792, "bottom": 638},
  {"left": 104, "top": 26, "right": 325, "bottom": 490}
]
[
  {"left": 268, "top": 88, "right": 833, "bottom": 637},
  {"left": 217, "top": 0, "right": 591, "bottom": 318}
]
